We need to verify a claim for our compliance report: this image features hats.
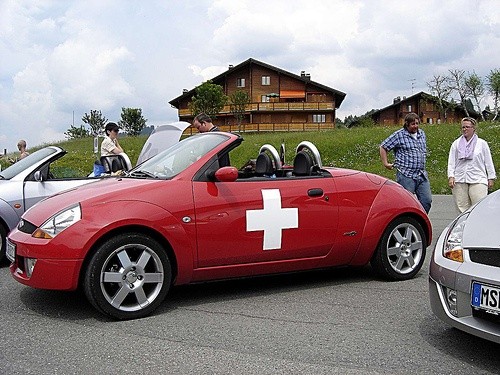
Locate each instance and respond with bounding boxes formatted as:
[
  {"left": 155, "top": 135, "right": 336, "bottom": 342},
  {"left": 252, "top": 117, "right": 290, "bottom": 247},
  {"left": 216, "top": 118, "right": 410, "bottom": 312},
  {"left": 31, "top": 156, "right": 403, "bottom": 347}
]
[{"left": 105, "top": 122, "right": 122, "bottom": 130}]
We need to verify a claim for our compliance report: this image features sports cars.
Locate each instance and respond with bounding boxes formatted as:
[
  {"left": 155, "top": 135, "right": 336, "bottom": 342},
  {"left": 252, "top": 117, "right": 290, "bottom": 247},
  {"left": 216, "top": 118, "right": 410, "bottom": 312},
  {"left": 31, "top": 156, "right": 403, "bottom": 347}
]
[
  {"left": 0, "top": 121, "right": 193, "bottom": 269},
  {"left": 5, "top": 130, "right": 434, "bottom": 321}
]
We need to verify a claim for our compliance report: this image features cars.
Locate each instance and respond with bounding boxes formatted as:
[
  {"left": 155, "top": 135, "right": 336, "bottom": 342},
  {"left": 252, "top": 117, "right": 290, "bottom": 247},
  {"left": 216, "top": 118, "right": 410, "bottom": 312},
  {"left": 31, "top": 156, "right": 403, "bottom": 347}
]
[{"left": 427, "top": 189, "right": 500, "bottom": 345}]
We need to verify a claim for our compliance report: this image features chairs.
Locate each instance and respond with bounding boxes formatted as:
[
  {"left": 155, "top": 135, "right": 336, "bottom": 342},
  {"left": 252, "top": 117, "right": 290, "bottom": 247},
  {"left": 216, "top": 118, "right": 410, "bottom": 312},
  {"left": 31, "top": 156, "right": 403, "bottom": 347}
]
[
  {"left": 112, "top": 159, "right": 123, "bottom": 173},
  {"left": 286, "top": 141, "right": 329, "bottom": 176},
  {"left": 255, "top": 144, "right": 284, "bottom": 177}
]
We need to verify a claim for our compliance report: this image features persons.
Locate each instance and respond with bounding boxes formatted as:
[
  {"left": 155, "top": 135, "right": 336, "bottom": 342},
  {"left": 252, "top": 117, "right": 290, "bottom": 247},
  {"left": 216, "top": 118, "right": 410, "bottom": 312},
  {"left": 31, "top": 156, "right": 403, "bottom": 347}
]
[
  {"left": 380, "top": 113, "right": 432, "bottom": 215},
  {"left": 447, "top": 117, "right": 497, "bottom": 213},
  {"left": 192, "top": 113, "right": 230, "bottom": 182},
  {"left": 101, "top": 122, "right": 124, "bottom": 174},
  {"left": 3, "top": 140, "right": 29, "bottom": 163}
]
